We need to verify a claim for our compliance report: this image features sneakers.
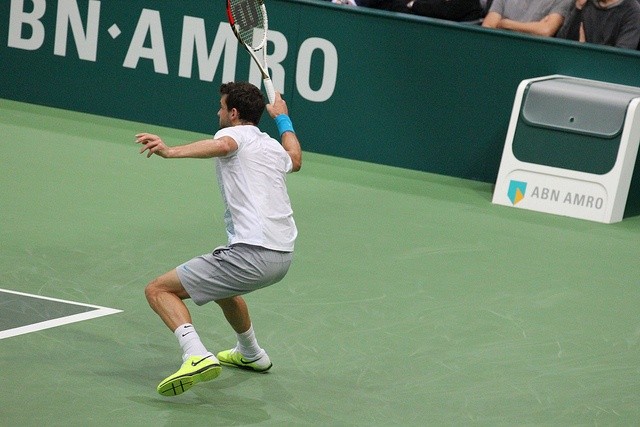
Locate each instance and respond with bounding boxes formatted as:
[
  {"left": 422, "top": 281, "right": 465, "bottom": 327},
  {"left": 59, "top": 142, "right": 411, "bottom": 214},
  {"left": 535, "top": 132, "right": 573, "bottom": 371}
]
[
  {"left": 157, "top": 352, "right": 221, "bottom": 397},
  {"left": 217, "top": 348, "right": 272, "bottom": 372}
]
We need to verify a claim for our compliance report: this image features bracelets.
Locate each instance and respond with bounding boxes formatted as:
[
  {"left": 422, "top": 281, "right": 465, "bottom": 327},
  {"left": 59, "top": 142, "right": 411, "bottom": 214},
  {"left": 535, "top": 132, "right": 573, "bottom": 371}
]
[{"left": 273, "top": 114, "right": 295, "bottom": 137}]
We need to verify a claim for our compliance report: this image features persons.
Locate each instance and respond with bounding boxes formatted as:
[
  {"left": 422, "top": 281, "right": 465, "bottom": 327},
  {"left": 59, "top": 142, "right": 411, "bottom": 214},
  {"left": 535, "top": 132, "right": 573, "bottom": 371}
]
[
  {"left": 135, "top": 81, "right": 302, "bottom": 397},
  {"left": 481, "top": 0, "right": 573, "bottom": 38},
  {"left": 556, "top": 0, "right": 640, "bottom": 50}
]
